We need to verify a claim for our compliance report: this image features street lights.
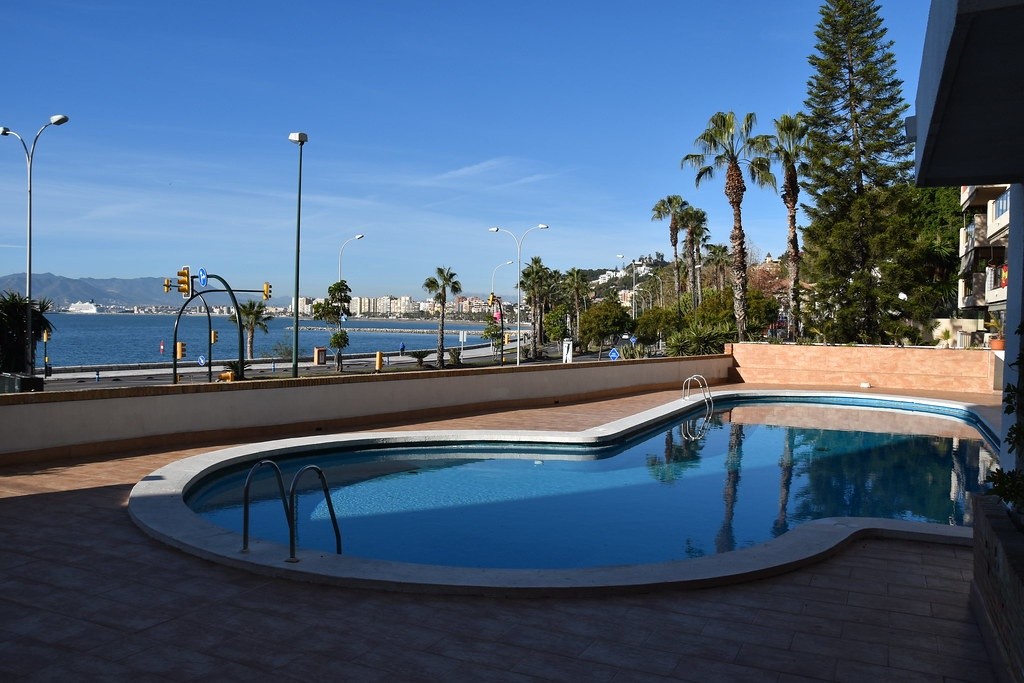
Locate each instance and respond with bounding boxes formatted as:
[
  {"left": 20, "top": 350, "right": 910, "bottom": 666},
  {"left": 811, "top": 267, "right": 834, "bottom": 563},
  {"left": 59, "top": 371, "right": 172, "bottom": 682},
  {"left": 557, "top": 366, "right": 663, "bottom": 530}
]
[
  {"left": 289, "top": 132, "right": 309, "bottom": 377},
  {"left": 0, "top": 114, "right": 70, "bottom": 375},
  {"left": 491, "top": 261, "right": 514, "bottom": 316},
  {"left": 338, "top": 235, "right": 364, "bottom": 329},
  {"left": 489, "top": 224, "right": 550, "bottom": 367},
  {"left": 616, "top": 254, "right": 664, "bottom": 352}
]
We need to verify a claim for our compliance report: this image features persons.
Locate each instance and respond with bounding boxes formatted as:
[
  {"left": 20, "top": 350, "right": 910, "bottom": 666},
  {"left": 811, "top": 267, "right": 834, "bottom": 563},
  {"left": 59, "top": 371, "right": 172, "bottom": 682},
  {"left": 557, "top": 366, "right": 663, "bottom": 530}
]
[{"left": 399, "top": 342, "right": 405, "bottom": 356}]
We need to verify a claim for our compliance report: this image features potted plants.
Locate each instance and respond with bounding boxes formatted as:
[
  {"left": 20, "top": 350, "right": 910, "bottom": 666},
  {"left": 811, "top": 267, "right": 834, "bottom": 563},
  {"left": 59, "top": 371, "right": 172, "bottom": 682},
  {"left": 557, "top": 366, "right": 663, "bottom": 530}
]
[{"left": 984, "top": 311, "right": 1005, "bottom": 350}]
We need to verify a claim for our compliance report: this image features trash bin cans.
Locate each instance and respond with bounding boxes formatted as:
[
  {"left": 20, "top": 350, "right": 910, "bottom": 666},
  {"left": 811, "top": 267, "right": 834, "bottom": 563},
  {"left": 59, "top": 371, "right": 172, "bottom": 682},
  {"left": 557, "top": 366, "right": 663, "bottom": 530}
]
[{"left": 314, "top": 346, "right": 327, "bottom": 364}]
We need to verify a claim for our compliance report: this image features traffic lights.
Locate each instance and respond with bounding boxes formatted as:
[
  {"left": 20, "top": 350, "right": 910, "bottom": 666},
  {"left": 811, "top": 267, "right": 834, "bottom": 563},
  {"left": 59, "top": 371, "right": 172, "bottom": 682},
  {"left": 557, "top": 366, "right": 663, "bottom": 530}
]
[
  {"left": 165, "top": 278, "right": 172, "bottom": 292},
  {"left": 263, "top": 282, "right": 272, "bottom": 300},
  {"left": 177, "top": 266, "right": 192, "bottom": 299},
  {"left": 43, "top": 330, "right": 51, "bottom": 343},
  {"left": 210, "top": 330, "right": 219, "bottom": 344},
  {"left": 177, "top": 342, "right": 186, "bottom": 360}
]
